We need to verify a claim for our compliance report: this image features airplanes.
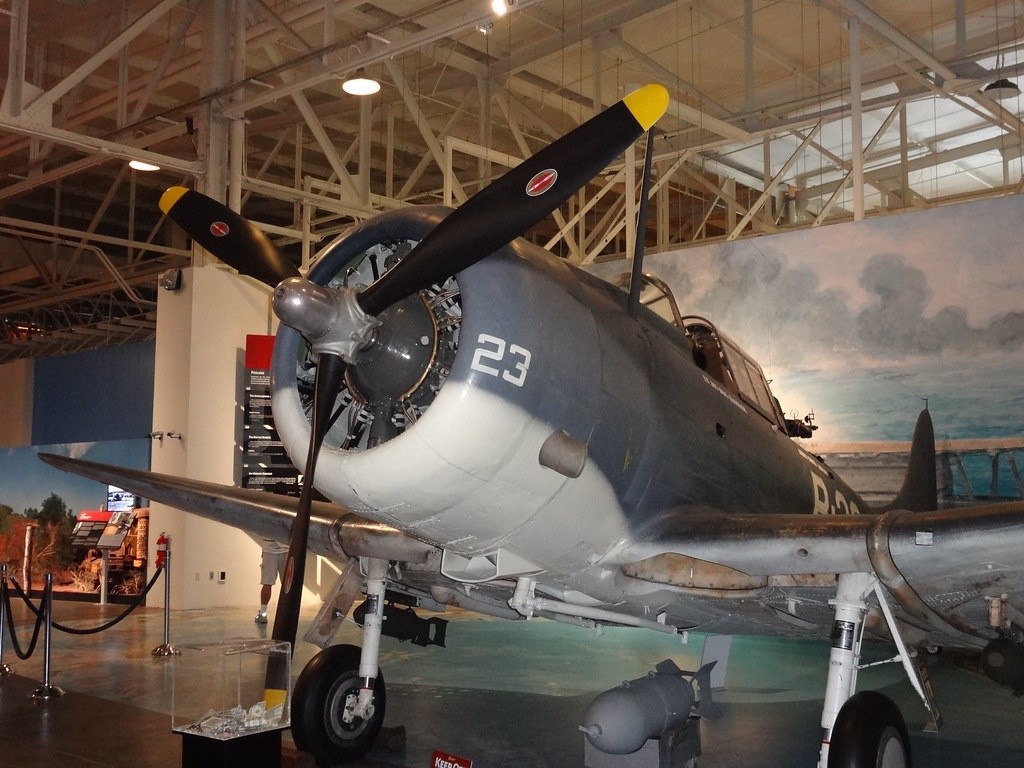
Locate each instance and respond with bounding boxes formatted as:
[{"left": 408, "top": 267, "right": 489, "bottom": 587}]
[{"left": 36, "top": 82, "right": 1023, "bottom": 767}]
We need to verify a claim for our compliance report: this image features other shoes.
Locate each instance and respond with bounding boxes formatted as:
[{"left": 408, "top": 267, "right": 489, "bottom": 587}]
[{"left": 255, "top": 610, "right": 267, "bottom": 623}]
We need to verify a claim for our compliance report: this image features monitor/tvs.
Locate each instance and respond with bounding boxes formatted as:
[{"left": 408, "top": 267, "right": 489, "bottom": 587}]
[{"left": 107, "top": 484, "right": 136, "bottom": 512}]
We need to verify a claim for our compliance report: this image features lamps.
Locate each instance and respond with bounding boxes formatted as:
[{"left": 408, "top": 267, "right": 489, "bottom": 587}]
[
  {"left": 129, "top": 147, "right": 160, "bottom": 172},
  {"left": 983, "top": 0, "right": 1022, "bottom": 99},
  {"left": 160, "top": 268, "right": 182, "bottom": 291},
  {"left": 492, "top": 0, "right": 519, "bottom": 17},
  {"left": 342, "top": 67, "right": 380, "bottom": 95}
]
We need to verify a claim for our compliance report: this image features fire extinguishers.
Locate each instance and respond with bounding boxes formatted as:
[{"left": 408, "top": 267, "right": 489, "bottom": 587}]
[{"left": 155, "top": 529, "right": 169, "bottom": 569}]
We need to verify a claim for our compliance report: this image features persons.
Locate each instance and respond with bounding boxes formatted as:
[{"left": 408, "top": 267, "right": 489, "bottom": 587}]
[{"left": 255, "top": 541, "right": 290, "bottom": 623}]
